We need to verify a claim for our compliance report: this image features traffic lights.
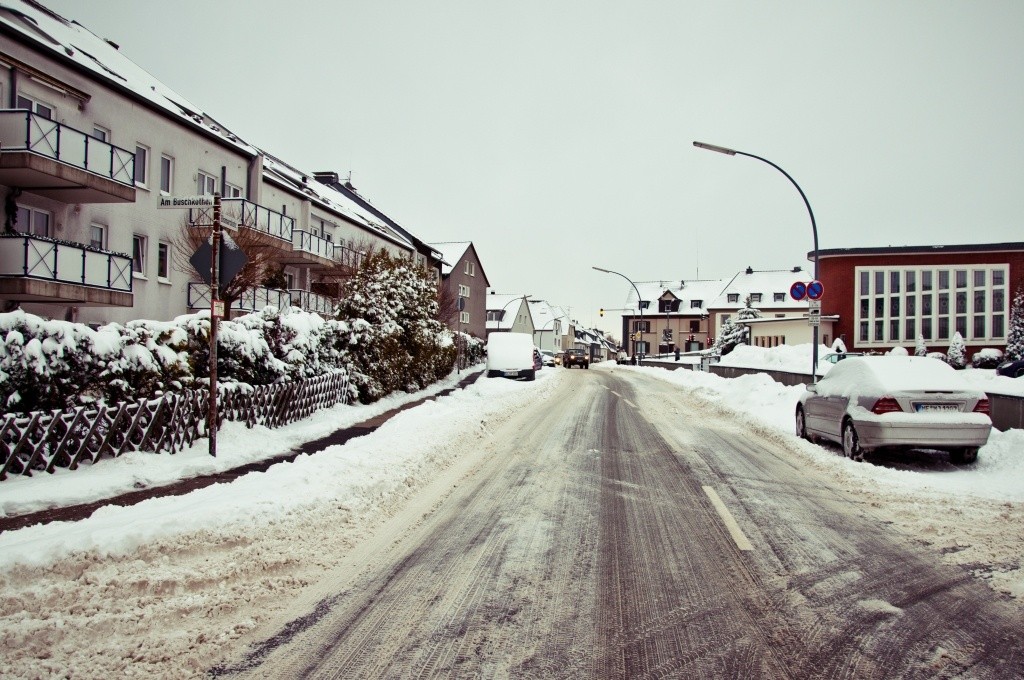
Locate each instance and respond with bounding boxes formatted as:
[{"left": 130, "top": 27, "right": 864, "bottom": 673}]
[
  {"left": 600, "top": 308, "right": 603, "bottom": 317},
  {"left": 689, "top": 334, "right": 696, "bottom": 342},
  {"left": 706, "top": 337, "right": 714, "bottom": 346},
  {"left": 667, "top": 333, "right": 673, "bottom": 341}
]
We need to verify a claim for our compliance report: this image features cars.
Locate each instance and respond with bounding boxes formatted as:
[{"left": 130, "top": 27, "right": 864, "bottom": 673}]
[
  {"left": 794, "top": 355, "right": 992, "bottom": 463},
  {"left": 554, "top": 353, "right": 561, "bottom": 365}
]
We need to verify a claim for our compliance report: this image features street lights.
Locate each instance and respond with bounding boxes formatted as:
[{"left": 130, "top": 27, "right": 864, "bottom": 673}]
[
  {"left": 540, "top": 315, "right": 564, "bottom": 349},
  {"left": 498, "top": 294, "right": 532, "bottom": 332},
  {"left": 667, "top": 298, "right": 677, "bottom": 359},
  {"left": 692, "top": 141, "right": 819, "bottom": 383},
  {"left": 592, "top": 266, "right": 642, "bottom": 366}
]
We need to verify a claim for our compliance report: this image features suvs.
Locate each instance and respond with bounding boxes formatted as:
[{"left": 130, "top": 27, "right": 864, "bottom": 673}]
[
  {"left": 538, "top": 350, "right": 555, "bottom": 367},
  {"left": 563, "top": 348, "right": 589, "bottom": 370},
  {"left": 533, "top": 348, "right": 542, "bottom": 370}
]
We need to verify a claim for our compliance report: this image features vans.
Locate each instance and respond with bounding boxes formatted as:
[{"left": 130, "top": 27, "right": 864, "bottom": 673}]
[{"left": 486, "top": 331, "right": 535, "bottom": 381}]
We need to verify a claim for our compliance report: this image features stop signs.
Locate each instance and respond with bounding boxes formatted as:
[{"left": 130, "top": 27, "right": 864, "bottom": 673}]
[
  {"left": 807, "top": 281, "right": 823, "bottom": 300},
  {"left": 790, "top": 281, "right": 807, "bottom": 300}
]
